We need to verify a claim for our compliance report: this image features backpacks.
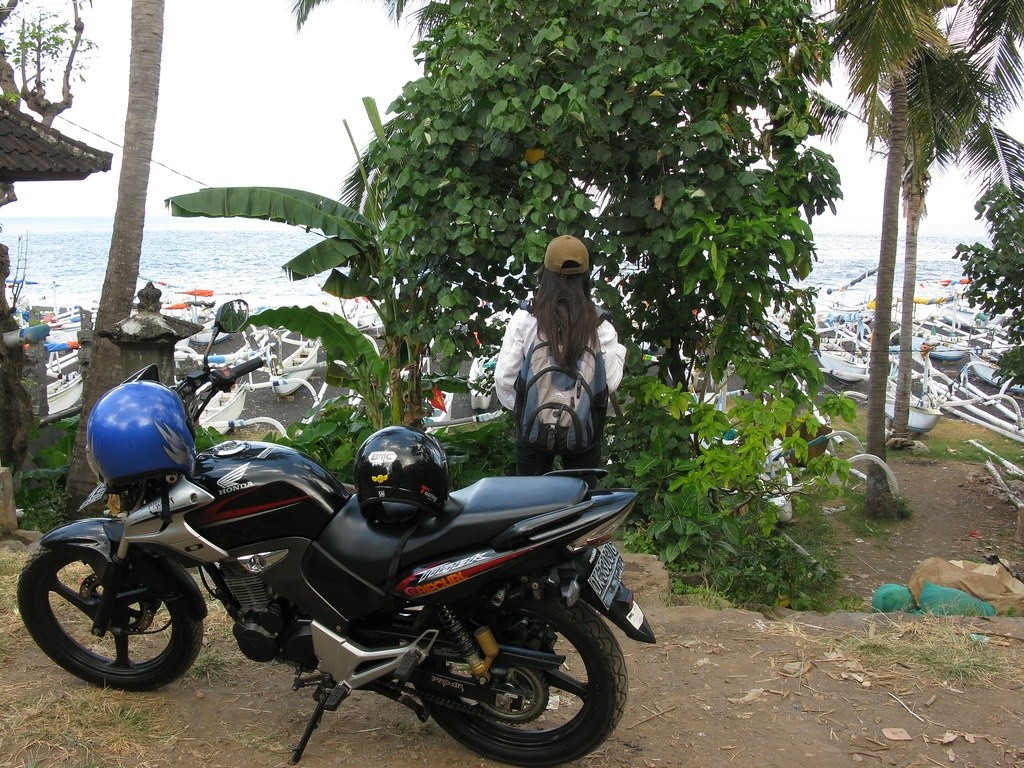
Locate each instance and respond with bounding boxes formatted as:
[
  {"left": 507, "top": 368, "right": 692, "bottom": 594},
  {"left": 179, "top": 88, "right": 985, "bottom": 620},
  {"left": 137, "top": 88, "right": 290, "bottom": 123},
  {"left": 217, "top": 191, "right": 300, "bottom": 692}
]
[{"left": 514, "top": 306, "right": 609, "bottom": 455}]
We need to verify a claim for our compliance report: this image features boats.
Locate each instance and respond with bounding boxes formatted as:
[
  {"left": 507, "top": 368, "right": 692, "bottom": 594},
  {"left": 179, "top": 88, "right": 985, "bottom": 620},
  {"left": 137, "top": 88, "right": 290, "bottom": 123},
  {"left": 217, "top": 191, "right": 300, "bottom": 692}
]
[
  {"left": 684, "top": 386, "right": 792, "bottom": 522},
  {"left": 742, "top": 299, "right": 1024, "bottom": 433},
  {"left": 46, "top": 318, "right": 318, "bottom": 440}
]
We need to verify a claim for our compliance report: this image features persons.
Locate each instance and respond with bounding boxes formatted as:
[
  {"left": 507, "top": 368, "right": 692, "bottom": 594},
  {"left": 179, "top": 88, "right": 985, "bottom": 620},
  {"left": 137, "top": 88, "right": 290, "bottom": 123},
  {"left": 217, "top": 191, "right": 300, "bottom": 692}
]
[{"left": 494, "top": 234, "right": 628, "bottom": 502}]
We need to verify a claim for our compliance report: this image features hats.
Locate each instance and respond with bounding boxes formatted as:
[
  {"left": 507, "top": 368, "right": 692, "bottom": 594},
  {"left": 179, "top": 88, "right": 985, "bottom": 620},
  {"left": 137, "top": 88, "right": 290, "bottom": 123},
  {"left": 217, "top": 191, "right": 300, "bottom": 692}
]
[{"left": 544, "top": 235, "right": 589, "bottom": 274}]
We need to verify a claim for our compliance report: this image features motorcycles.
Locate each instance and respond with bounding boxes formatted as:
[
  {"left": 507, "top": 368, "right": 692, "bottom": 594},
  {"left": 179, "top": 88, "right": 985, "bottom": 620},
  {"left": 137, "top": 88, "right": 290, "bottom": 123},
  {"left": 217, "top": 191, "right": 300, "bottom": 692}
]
[{"left": 17, "top": 300, "right": 657, "bottom": 767}]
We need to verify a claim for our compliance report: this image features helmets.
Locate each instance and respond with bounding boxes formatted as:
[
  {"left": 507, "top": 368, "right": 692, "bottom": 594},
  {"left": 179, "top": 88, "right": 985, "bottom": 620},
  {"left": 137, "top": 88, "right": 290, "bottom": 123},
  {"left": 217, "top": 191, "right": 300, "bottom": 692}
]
[
  {"left": 87, "top": 381, "right": 197, "bottom": 491},
  {"left": 354, "top": 425, "right": 451, "bottom": 518}
]
